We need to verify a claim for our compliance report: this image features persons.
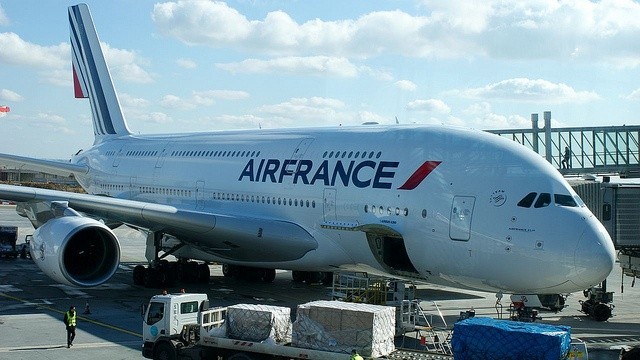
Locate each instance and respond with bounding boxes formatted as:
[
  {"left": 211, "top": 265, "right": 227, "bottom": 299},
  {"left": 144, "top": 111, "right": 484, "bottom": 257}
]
[
  {"left": 158, "top": 306, "right": 164, "bottom": 319},
  {"left": 64, "top": 305, "right": 77, "bottom": 348},
  {"left": 350, "top": 348, "right": 364, "bottom": 360}
]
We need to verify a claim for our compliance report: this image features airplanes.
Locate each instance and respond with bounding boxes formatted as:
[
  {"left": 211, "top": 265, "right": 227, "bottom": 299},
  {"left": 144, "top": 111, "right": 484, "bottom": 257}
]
[{"left": 1, "top": 3, "right": 617, "bottom": 294}]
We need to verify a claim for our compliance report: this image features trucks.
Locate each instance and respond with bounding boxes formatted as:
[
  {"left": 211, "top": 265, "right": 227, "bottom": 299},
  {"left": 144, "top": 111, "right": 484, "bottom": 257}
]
[{"left": 142, "top": 288, "right": 454, "bottom": 360}]
